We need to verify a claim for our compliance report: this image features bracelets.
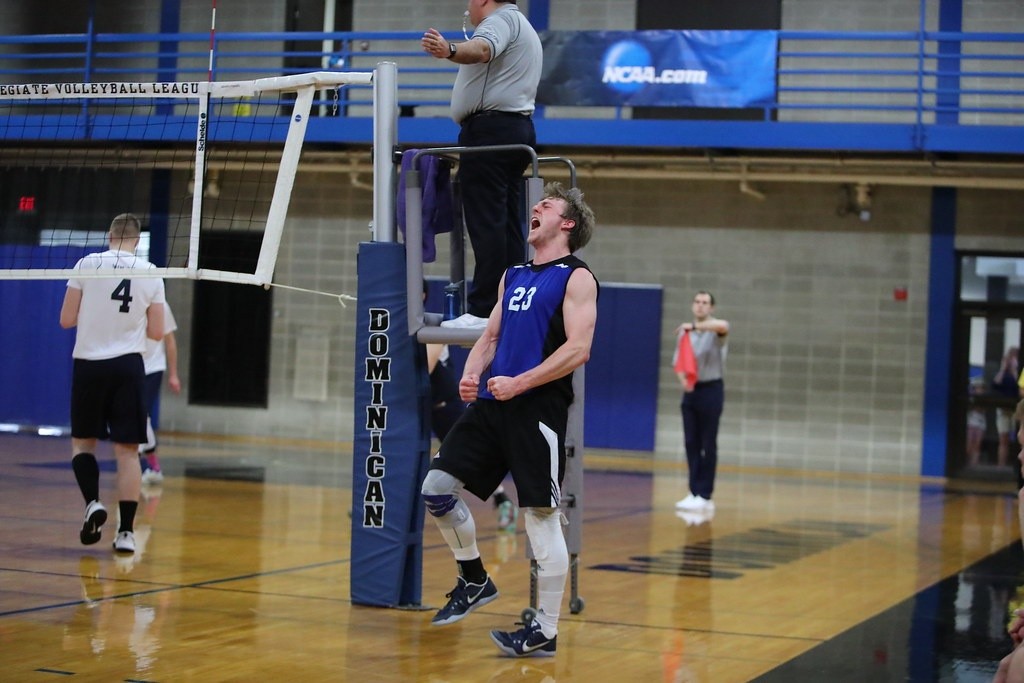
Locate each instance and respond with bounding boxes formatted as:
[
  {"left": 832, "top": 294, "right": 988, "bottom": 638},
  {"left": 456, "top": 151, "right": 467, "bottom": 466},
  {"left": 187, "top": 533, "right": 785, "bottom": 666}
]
[{"left": 692, "top": 322, "right": 695, "bottom": 330}]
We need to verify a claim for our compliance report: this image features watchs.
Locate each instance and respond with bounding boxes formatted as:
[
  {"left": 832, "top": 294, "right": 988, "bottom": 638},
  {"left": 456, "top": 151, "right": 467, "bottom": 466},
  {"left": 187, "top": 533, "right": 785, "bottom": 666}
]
[{"left": 446, "top": 43, "right": 456, "bottom": 59}]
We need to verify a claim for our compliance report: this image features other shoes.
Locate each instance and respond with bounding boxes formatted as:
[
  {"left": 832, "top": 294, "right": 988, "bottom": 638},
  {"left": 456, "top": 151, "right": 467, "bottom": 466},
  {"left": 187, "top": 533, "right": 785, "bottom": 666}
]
[
  {"left": 141, "top": 466, "right": 163, "bottom": 483},
  {"left": 674, "top": 494, "right": 695, "bottom": 509},
  {"left": 497, "top": 502, "right": 518, "bottom": 533},
  {"left": 677, "top": 512, "right": 712, "bottom": 526},
  {"left": 685, "top": 495, "right": 713, "bottom": 510}
]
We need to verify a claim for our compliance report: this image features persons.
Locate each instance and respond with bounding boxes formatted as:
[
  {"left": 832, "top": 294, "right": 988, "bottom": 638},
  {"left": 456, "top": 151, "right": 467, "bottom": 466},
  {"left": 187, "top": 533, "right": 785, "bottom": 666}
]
[
  {"left": 671, "top": 291, "right": 729, "bottom": 512},
  {"left": 969, "top": 347, "right": 1024, "bottom": 683},
  {"left": 419, "top": 181, "right": 601, "bottom": 657},
  {"left": 421, "top": 0, "right": 542, "bottom": 330},
  {"left": 60, "top": 214, "right": 180, "bottom": 554}
]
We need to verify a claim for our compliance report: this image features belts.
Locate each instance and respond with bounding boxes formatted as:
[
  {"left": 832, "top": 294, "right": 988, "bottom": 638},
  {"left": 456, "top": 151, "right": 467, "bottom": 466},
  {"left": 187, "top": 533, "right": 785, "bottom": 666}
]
[{"left": 470, "top": 110, "right": 523, "bottom": 117}]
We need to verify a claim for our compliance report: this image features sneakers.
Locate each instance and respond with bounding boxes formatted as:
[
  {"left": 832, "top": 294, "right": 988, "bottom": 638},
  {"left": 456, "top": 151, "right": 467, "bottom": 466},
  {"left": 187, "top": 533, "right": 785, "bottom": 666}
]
[
  {"left": 490, "top": 617, "right": 557, "bottom": 658},
  {"left": 113, "top": 531, "right": 133, "bottom": 551},
  {"left": 80, "top": 501, "right": 107, "bottom": 545},
  {"left": 441, "top": 311, "right": 489, "bottom": 330},
  {"left": 432, "top": 574, "right": 498, "bottom": 626}
]
